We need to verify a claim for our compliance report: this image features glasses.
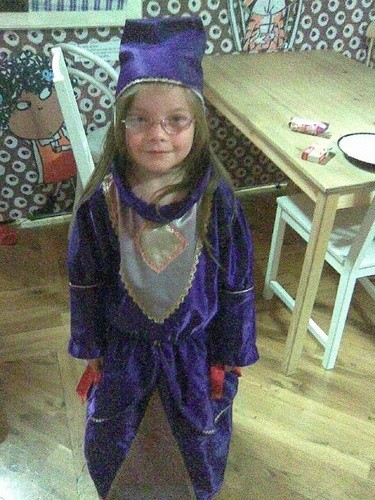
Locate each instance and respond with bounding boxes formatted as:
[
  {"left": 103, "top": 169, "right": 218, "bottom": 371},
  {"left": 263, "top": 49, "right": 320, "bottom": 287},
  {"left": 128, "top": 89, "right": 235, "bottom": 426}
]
[{"left": 119, "top": 113, "right": 194, "bottom": 131}]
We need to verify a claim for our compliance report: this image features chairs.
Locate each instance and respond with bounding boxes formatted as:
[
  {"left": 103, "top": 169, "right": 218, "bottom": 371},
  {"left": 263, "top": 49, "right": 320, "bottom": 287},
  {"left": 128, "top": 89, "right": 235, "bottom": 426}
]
[
  {"left": 51, "top": 46, "right": 95, "bottom": 241},
  {"left": 262, "top": 192, "right": 375, "bottom": 370}
]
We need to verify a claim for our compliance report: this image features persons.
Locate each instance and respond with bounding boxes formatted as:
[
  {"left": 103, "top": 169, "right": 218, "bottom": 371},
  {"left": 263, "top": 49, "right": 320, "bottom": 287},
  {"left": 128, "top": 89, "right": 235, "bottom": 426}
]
[{"left": 66, "top": 15, "right": 260, "bottom": 500}]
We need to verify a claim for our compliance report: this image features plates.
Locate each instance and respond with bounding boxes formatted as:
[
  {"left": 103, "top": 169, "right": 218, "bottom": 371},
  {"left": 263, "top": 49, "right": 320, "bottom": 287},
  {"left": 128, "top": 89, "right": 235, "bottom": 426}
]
[{"left": 337, "top": 132, "right": 375, "bottom": 168}]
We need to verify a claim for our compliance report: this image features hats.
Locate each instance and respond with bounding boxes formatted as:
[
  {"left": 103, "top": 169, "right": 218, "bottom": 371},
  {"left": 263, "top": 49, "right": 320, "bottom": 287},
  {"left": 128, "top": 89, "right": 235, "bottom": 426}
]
[{"left": 115, "top": 17, "right": 207, "bottom": 107}]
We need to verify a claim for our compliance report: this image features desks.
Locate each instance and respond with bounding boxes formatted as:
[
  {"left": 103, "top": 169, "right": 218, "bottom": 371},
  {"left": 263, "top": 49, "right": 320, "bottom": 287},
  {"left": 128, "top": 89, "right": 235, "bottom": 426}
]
[{"left": 200, "top": 49, "right": 375, "bottom": 376}]
